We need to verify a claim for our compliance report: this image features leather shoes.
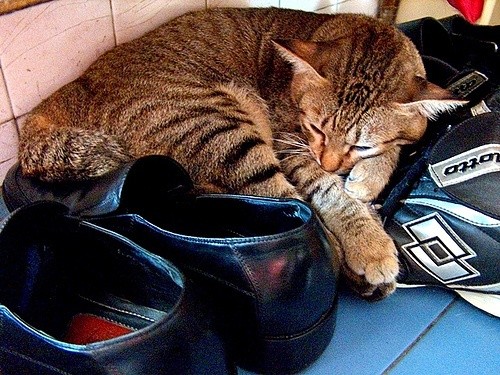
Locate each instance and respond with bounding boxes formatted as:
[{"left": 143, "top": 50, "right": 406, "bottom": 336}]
[
  {"left": 2, "top": 154, "right": 337, "bottom": 373},
  {"left": 1, "top": 199, "right": 237, "bottom": 374},
  {"left": 396, "top": 17, "right": 498, "bottom": 103},
  {"left": 439, "top": 14, "right": 500, "bottom": 48}
]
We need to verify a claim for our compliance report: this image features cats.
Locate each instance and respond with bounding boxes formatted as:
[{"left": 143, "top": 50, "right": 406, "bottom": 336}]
[{"left": 15, "top": 5, "right": 472, "bottom": 286}]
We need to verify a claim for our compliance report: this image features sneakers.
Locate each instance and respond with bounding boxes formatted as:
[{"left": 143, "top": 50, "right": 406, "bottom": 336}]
[{"left": 377, "top": 103, "right": 499, "bottom": 319}]
